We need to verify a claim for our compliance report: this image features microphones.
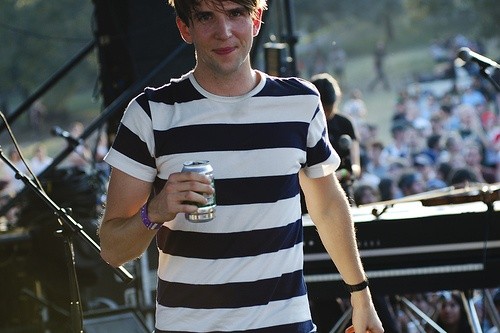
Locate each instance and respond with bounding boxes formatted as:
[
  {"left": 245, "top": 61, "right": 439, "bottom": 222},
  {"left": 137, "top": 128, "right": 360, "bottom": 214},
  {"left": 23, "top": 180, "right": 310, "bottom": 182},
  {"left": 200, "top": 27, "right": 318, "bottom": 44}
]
[
  {"left": 457, "top": 46, "right": 500, "bottom": 72},
  {"left": 339, "top": 135, "right": 352, "bottom": 176},
  {"left": 51, "top": 126, "right": 84, "bottom": 146}
]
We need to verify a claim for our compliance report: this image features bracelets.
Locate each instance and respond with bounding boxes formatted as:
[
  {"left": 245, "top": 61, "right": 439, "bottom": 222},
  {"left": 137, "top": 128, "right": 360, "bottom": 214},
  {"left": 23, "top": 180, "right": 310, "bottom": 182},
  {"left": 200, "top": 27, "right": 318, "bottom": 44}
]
[
  {"left": 348, "top": 280, "right": 369, "bottom": 292},
  {"left": 141, "top": 199, "right": 164, "bottom": 230}
]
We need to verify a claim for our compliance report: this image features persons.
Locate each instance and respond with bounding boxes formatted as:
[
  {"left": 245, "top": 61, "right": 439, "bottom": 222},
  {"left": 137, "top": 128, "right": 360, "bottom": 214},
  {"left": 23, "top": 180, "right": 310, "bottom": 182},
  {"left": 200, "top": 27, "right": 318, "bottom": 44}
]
[
  {"left": 308, "top": 30, "right": 500, "bottom": 206},
  {"left": 100, "top": 0, "right": 384, "bottom": 333},
  {"left": 300, "top": 73, "right": 361, "bottom": 213},
  {"left": 0, "top": 121, "right": 108, "bottom": 232}
]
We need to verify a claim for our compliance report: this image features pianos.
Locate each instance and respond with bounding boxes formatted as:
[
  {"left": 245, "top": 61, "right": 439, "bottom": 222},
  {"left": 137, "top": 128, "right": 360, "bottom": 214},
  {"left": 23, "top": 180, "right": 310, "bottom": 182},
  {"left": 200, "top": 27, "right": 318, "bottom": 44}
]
[{"left": 302, "top": 182, "right": 500, "bottom": 333}]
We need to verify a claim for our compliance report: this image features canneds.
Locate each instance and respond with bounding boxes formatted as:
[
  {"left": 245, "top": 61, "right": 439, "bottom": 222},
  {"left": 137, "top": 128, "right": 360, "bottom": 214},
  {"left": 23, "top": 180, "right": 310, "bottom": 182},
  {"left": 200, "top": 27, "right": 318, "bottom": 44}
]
[{"left": 180, "top": 160, "right": 217, "bottom": 223}]
